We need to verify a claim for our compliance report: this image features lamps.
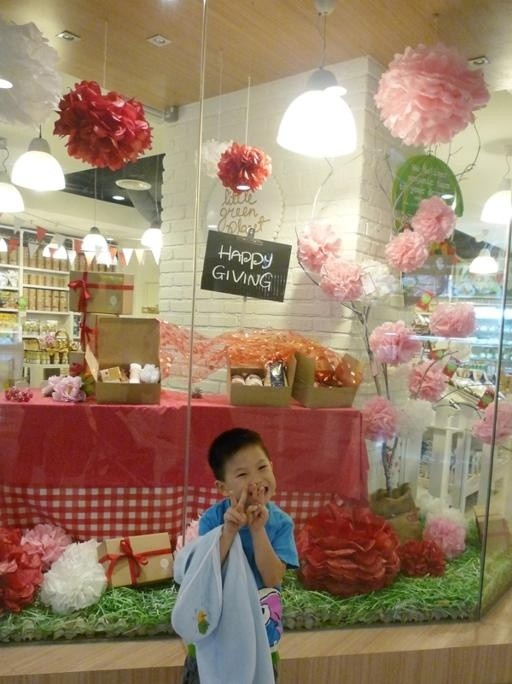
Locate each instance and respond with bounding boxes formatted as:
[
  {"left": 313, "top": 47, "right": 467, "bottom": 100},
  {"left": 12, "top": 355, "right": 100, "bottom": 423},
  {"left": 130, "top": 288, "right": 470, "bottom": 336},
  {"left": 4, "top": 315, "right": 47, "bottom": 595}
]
[{"left": 479, "top": 152, "right": 512, "bottom": 224}]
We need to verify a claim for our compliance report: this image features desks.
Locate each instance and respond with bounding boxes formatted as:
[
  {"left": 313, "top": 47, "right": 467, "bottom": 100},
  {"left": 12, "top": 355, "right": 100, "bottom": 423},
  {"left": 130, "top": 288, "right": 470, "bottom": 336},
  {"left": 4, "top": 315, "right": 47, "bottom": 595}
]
[{"left": 1, "top": 389, "right": 365, "bottom": 544}]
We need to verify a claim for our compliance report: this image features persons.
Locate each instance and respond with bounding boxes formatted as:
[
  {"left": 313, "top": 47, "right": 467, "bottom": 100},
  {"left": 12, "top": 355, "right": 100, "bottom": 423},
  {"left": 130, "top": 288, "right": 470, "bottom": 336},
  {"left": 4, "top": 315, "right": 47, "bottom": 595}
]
[{"left": 174, "top": 427, "right": 300, "bottom": 683}]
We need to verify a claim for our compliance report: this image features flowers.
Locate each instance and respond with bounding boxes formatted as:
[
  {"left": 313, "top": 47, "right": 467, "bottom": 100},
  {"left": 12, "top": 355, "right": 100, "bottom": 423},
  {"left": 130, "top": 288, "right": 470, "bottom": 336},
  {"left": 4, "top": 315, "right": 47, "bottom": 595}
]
[
  {"left": 41, "top": 375, "right": 85, "bottom": 402},
  {"left": 1, "top": 524, "right": 108, "bottom": 614},
  {"left": 5, "top": 386, "right": 33, "bottom": 402},
  {"left": 371, "top": 12, "right": 490, "bottom": 149}
]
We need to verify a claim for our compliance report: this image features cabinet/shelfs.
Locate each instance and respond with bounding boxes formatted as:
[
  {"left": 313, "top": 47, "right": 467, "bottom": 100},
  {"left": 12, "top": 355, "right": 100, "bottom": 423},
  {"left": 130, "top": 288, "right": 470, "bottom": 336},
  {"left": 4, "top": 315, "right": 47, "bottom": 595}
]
[{"left": 0, "top": 225, "right": 119, "bottom": 385}]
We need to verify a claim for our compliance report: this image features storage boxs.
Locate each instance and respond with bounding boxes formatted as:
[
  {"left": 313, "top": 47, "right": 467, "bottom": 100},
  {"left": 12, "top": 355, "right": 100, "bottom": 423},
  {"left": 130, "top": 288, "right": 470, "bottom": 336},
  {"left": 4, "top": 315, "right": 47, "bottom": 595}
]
[
  {"left": 97, "top": 532, "right": 174, "bottom": 587},
  {"left": 225, "top": 353, "right": 363, "bottom": 408},
  {"left": 68, "top": 269, "right": 160, "bottom": 404}
]
